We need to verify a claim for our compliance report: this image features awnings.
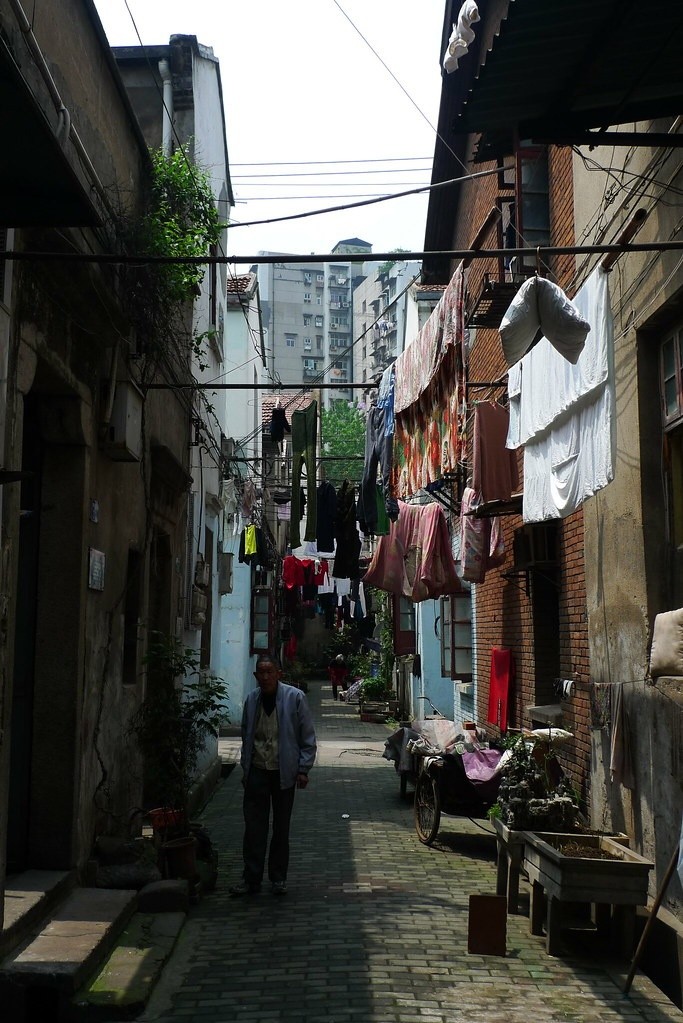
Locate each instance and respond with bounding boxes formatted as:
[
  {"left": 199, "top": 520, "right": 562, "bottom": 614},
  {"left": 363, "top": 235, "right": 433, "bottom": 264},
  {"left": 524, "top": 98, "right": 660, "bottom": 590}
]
[{"left": 459, "top": 0, "right": 683, "bottom": 164}]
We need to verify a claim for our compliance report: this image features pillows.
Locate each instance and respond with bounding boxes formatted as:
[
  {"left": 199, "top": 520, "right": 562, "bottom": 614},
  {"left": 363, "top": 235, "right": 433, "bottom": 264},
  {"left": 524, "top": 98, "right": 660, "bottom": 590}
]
[
  {"left": 650, "top": 607, "right": 683, "bottom": 677},
  {"left": 498, "top": 275, "right": 592, "bottom": 367}
]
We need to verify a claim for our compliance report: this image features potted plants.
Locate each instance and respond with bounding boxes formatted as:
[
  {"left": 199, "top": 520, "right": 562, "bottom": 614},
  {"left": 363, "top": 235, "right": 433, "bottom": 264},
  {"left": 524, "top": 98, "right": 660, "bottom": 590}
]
[
  {"left": 289, "top": 590, "right": 402, "bottom": 721},
  {"left": 488, "top": 728, "right": 588, "bottom": 914},
  {"left": 521, "top": 830, "right": 656, "bottom": 955}
]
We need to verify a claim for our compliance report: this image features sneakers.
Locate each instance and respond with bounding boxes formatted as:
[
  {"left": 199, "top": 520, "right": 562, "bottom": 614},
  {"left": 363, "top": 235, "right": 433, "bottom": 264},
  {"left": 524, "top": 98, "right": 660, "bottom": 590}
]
[
  {"left": 229, "top": 879, "right": 264, "bottom": 894},
  {"left": 271, "top": 880, "right": 289, "bottom": 893}
]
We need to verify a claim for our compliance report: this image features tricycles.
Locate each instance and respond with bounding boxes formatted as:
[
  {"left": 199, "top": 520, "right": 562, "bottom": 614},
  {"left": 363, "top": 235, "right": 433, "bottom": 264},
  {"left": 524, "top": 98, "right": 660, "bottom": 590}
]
[{"left": 397, "top": 695, "right": 510, "bottom": 844}]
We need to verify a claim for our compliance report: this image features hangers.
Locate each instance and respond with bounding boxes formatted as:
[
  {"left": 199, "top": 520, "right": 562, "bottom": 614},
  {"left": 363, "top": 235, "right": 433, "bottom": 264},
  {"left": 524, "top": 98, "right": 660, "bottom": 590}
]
[{"left": 221, "top": 246, "right": 546, "bottom": 607}]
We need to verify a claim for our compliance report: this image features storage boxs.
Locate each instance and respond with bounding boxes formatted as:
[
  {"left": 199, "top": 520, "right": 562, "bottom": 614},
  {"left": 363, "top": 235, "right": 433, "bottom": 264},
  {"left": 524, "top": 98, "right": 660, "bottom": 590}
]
[{"left": 467, "top": 894, "right": 508, "bottom": 958}]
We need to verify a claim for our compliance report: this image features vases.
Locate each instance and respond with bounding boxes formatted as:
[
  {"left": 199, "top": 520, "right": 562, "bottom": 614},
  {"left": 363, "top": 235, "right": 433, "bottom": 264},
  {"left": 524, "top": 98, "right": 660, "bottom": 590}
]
[{"left": 123, "top": 640, "right": 228, "bottom": 880}]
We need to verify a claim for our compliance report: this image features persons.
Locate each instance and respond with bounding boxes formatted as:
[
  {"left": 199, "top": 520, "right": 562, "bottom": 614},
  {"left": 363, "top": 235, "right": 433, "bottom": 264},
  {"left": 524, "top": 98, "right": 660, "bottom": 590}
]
[
  {"left": 328, "top": 654, "right": 350, "bottom": 701},
  {"left": 230, "top": 656, "right": 316, "bottom": 899}
]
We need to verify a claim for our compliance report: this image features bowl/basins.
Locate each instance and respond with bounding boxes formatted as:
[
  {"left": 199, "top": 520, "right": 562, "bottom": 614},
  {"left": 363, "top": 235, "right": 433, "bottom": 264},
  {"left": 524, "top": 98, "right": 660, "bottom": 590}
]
[
  {"left": 377, "top": 715, "right": 388, "bottom": 722},
  {"left": 362, "top": 705, "right": 379, "bottom": 712}
]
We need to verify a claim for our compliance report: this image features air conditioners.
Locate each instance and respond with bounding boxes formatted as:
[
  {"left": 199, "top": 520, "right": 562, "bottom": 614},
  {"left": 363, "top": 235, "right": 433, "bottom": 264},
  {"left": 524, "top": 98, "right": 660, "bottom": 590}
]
[
  {"left": 343, "top": 302, "right": 349, "bottom": 307},
  {"left": 220, "top": 438, "right": 234, "bottom": 457},
  {"left": 195, "top": 560, "right": 210, "bottom": 585},
  {"left": 512, "top": 523, "right": 559, "bottom": 567},
  {"left": 330, "top": 345, "right": 337, "bottom": 351},
  {"left": 331, "top": 323, "right": 337, "bottom": 328}
]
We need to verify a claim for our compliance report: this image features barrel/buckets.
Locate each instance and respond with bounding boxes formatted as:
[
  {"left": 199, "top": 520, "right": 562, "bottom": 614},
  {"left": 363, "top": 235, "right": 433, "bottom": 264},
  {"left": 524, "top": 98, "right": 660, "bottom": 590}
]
[
  {"left": 148, "top": 806, "right": 184, "bottom": 828},
  {"left": 222, "top": 762, "right": 236, "bottom": 778},
  {"left": 161, "top": 837, "right": 197, "bottom": 878}
]
